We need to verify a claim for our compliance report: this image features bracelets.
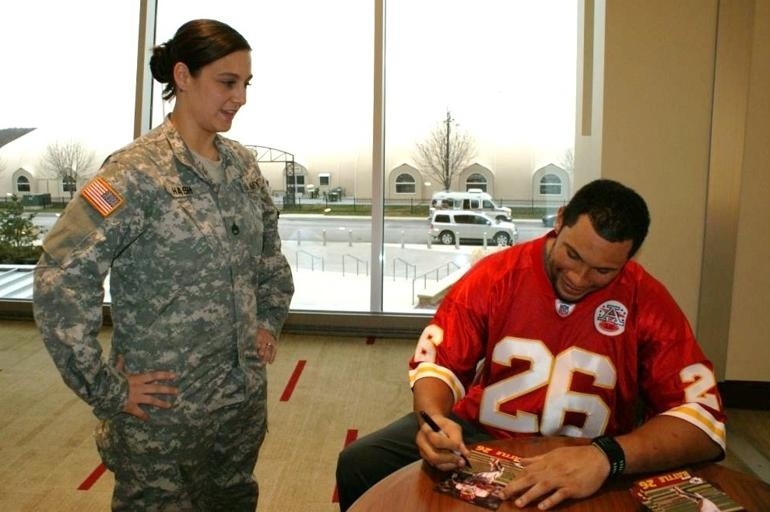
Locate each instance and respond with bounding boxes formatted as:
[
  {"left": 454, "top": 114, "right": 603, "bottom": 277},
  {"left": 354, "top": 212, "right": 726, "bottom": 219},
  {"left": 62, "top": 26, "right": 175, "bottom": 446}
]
[{"left": 591, "top": 433, "right": 625, "bottom": 484}]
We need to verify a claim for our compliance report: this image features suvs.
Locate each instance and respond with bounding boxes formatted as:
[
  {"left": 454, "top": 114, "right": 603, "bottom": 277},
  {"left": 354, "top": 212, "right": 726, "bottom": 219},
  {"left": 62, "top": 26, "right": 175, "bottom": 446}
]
[{"left": 433, "top": 188, "right": 514, "bottom": 221}]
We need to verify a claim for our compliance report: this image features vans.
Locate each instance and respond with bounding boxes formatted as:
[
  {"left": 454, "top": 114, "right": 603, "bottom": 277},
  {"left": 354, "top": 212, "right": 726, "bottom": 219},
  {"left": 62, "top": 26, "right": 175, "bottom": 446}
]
[{"left": 430, "top": 208, "right": 518, "bottom": 245}]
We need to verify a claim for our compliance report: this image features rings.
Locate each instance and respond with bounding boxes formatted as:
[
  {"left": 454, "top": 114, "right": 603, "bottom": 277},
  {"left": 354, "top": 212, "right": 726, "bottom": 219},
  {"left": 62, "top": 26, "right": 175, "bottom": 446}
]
[{"left": 266, "top": 342, "right": 273, "bottom": 347}]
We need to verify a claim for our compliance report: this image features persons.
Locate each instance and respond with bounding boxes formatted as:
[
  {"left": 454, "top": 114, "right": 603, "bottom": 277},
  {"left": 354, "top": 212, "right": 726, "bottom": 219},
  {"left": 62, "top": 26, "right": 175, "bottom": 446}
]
[
  {"left": 336, "top": 177, "right": 728, "bottom": 512},
  {"left": 669, "top": 484, "right": 722, "bottom": 512},
  {"left": 452, "top": 458, "right": 504, "bottom": 504},
  {"left": 30, "top": 18, "right": 295, "bottom": 512}
]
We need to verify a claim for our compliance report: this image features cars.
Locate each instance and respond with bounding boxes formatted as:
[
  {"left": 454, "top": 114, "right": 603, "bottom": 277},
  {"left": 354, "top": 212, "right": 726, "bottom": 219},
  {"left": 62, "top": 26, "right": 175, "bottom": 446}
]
[{"left": 542, "top": 214, "right": 557, "bottom": 228}]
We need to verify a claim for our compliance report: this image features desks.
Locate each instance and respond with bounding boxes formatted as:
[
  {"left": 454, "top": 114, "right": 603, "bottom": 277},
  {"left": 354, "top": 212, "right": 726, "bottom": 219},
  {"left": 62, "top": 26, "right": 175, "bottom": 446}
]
[{"left": 346, "top": 435, "right": 770, "bottom": 511}]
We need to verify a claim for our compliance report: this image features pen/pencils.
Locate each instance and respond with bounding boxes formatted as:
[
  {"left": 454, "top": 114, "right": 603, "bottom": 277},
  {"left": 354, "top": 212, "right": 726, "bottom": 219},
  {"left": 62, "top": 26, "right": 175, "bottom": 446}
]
[{"left": 420, "top": 411, "right": 473, "bottom": 469}]
